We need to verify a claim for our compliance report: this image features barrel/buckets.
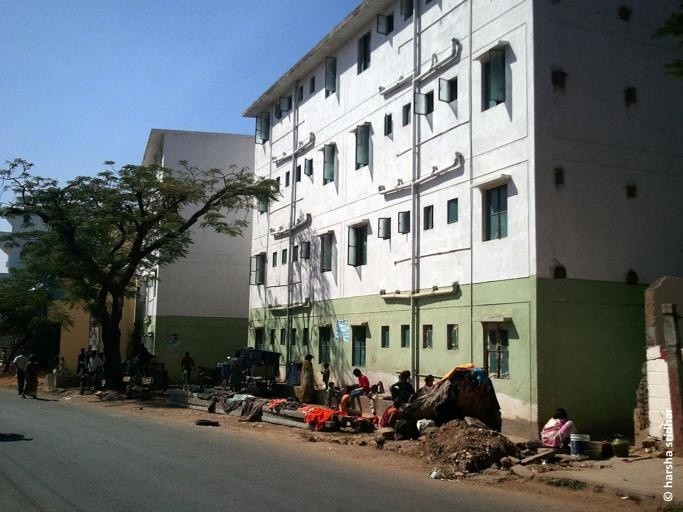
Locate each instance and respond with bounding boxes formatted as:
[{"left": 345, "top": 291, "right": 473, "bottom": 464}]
[
  {"left": 584, "top": 440, "right": 604, "bottom": 460},
  {"left": 570, "top": 433, "right": 591, "bottom": 459}
]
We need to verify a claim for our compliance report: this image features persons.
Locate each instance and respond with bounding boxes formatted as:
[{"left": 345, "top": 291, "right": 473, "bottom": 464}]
[
  {"left": 227, "top": 349, "right": 242, "bottom": 392},
  {"left": 540, "top": 408, "right": 574, "bottom": 447},
  {"left": 0, "top": 343, "right": 106, "bottom": 402},
  {"left": 296, "top": 353, "right": 436, "bottom": 428},
  {"left": 135, "top": 346, "right": 157, "bottom": 378},
  {"left": 179, "top": 351, "right": 196, "bottom": 385}
]
